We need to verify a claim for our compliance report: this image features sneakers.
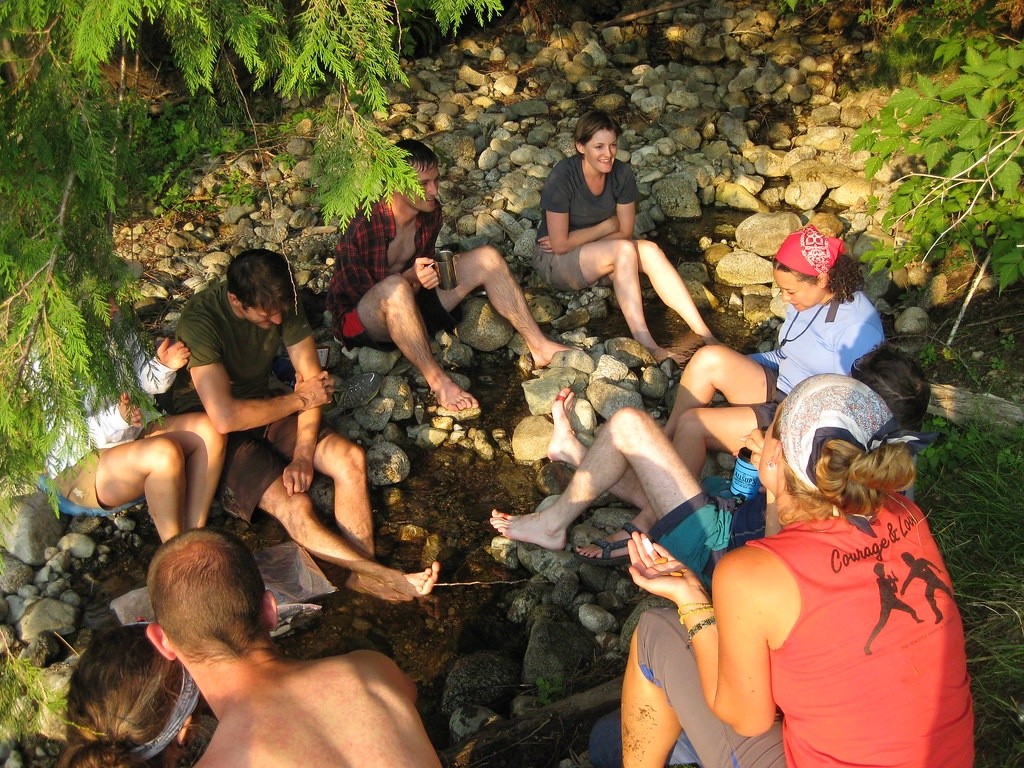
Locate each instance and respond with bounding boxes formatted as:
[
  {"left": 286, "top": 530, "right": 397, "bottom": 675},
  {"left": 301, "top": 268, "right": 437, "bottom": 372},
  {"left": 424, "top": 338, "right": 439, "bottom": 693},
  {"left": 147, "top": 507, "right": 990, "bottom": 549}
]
[{"left": 322, "top": 371, "right": 382, "bottom": 419}]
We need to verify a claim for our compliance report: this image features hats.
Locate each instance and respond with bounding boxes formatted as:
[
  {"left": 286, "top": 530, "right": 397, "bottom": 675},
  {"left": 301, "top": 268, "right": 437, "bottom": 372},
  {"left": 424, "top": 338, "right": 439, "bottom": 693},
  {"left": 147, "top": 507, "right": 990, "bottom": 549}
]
[{"left": 65, "top": 250, "right": 144, "bottom": 295}]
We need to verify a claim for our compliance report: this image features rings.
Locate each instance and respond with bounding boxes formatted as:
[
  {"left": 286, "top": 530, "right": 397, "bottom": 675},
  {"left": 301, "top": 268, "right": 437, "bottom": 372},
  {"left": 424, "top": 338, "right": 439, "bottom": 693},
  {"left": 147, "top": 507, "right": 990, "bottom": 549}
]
[{"left": 301, "top": 486, "right": 305, "bottom": 488}]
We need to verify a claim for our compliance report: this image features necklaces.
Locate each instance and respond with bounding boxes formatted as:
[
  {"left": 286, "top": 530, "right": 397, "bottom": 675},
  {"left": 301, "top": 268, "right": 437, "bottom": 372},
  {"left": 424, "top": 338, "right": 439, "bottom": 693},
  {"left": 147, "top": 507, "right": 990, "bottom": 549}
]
[{"left": 775, "top": 297, "right": 833, "bottom": 359}]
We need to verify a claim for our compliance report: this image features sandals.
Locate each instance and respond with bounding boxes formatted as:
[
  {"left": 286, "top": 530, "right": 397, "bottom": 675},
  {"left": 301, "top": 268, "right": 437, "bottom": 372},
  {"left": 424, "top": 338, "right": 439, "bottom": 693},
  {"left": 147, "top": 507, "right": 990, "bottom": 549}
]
[{"left": 573, "top": 522, "right": 655, "bottom": 567}]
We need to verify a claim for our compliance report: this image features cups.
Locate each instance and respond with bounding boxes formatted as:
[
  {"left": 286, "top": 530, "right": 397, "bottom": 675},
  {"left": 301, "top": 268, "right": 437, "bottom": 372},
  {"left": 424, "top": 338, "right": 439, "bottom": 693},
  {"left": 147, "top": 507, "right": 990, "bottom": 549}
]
[
  {"left": 428, "top": 250, "right": 459, "bottom": 290},
  {"left": 729, "top": 447, "right": 762, "bottom": 501}
]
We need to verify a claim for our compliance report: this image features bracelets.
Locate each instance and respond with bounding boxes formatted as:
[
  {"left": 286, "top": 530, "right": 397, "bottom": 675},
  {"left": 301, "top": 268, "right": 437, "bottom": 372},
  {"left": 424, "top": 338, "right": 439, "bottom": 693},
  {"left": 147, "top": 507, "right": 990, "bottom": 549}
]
[
  {"left": 678, "top": 603, "right": 713, "bottom": 624},
  {"left": 685, "top": 616, "right": 715, "bottom": 649}
]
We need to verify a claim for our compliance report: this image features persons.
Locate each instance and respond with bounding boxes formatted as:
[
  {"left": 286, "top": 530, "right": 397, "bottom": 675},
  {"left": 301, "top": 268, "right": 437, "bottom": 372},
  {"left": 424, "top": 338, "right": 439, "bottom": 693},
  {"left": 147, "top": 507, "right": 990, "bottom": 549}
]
[
  {"left": 489, "top": 340, "right": 931, "bottom": 585},
  {"left": 52, "top": 622, "right": 201, "bottom": 768},
  {"left": 23, "top": 255, "right": 227, "bottom": 545},
  {"left": 327, "top": 139, "right": 572, "bottom": 412},
  {"left": 153, "top": 249, "right": 375, "bottom": 559},
  {"left": 146, "top": 526, "right": 443, "bottom": 768},
  {"left": 575, "top": 223, "right": 885, "bottom": 567},
  {"left": 532, "top": 109, "right": 714, "bottom": 358},
  {"left": 621, "top": 372, "right": 977, "bottom": 768}
]
